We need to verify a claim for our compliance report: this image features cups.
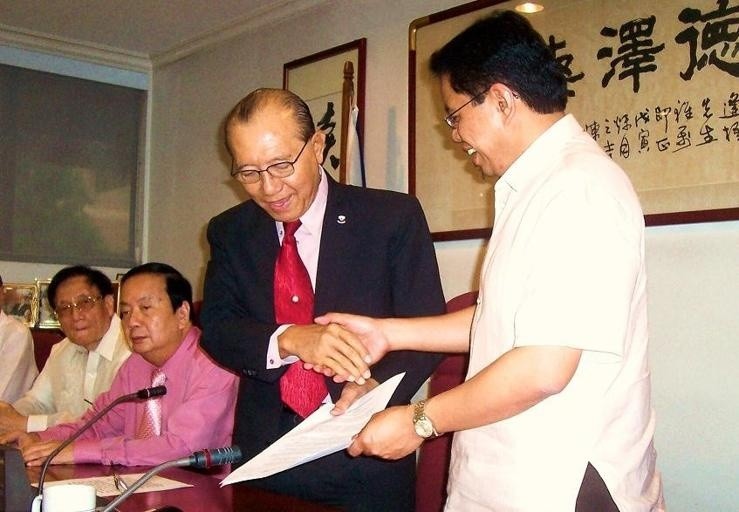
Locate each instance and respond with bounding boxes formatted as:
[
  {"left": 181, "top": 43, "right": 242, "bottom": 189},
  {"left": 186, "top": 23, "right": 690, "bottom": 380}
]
[{"left": 31, "top": 483, "right": 99, "bottom": 512}]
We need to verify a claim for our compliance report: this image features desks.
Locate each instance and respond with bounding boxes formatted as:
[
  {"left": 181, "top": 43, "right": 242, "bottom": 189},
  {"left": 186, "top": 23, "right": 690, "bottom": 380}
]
[{"left": 32, "top": 454, "right": 349, "bottom": 510}]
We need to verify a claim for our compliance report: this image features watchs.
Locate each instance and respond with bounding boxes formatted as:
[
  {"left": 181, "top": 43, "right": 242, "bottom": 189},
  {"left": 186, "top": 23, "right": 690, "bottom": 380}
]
[{"left": 412, "top": 400, "right": 445, "bottom": 440}]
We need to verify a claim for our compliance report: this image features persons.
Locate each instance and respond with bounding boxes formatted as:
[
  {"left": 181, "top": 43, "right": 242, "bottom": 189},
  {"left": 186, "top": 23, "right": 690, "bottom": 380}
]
[
  {"left": 0, "top": 277, "right": 40, "bottom": 405},
  {"left": 303, "top": 9, "right": 664, "bottom": 512},
  {"left": 0, "top": 265, "right": 132, "bottom": 448},
  {"left": 16, "top": 263, "right": 240, "bottom": 481},
  {"left": 197, "top": 88, "right": 446, "bottom": 511}
]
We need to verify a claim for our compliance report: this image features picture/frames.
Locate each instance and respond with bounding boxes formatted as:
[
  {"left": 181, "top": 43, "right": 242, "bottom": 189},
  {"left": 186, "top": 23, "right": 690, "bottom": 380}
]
[
  {"left": 34, "top": 278, "right": 65, "bottom": 329},
  {"left": 1, "top": 282, "right": 40, "bottom": 327},
  {"left": 281, "top": 37, "right": 365, "bottom": 191},
  {"left": 408, "top": 0, "right": 739, "bottom": 243},
  {"left": 110, "top": 279, "right": 122, "bottom": 318}
]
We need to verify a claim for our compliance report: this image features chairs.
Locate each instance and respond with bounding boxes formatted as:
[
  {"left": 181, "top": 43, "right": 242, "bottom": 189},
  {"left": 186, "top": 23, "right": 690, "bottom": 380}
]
[{"left": 412, "top": 287, "right": 479, "bottom": 512}]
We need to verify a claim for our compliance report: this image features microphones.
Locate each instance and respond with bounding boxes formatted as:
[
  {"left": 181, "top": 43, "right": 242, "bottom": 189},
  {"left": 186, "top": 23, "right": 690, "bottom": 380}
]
[
  {"left": 0, "top": 385, "right": 167, "bottom": 512},
  {"left": 100, "top": 445, "right": 242, "bottom": 511}
]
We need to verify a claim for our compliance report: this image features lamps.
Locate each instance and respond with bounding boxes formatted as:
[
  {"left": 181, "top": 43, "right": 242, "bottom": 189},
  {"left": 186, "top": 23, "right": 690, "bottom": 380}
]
[{"left": 514, "top": 1, "right": 544, "bottom": 14}]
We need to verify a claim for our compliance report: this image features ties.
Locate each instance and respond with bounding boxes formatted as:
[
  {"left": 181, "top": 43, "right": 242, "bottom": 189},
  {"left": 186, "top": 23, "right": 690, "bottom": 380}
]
[
  {"left": 135, "top": 370, "right": 166, "bottom": 439},
  {"left": 273, "top": 219, "right": 329, "bottom": 419}
]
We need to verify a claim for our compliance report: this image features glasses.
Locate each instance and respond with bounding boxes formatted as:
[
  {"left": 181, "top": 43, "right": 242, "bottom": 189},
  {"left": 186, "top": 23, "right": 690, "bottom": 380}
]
[
  {"left": 443, "top": 80, "right": 519, "bottom": 127},
  {"left": 230, "top": 135, "right": 312, "bottom": 185},
  {"left": 54, "top": 291, "right": 106, "bottom": 317},
  {"left": 107, "top": 459, "right": 130, "bottom": 492}
]
